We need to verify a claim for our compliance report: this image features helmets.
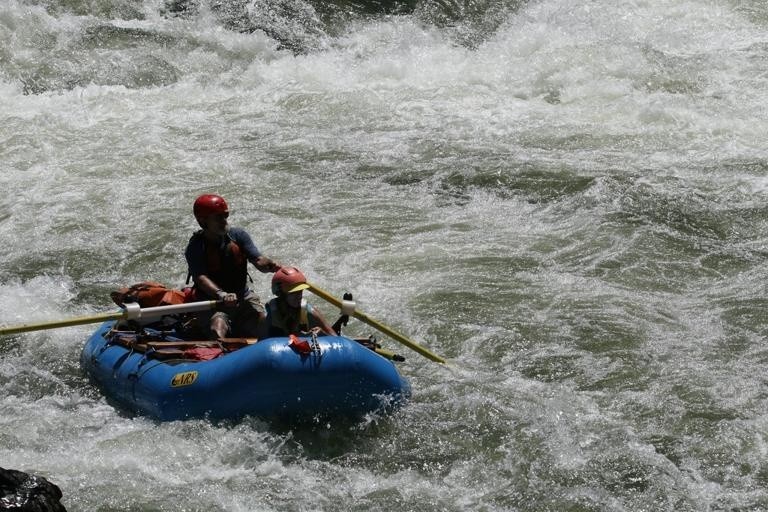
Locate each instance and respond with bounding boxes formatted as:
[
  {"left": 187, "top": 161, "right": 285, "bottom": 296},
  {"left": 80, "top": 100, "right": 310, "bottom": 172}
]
[
  {"left": 193, "top": 194, "right": 229, "bottom": 227},
  {"left": 272, "top": 267, "right": 308, "bottom": 295}
]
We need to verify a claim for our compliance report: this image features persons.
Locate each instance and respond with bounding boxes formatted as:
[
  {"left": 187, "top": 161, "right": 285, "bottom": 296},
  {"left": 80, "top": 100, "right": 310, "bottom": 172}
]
[
  {"left": 268, "top": 268, "right": 339, "bottom": 336},
  {"left": 185, "top": 194, "right": 273, "bottom": 348}
]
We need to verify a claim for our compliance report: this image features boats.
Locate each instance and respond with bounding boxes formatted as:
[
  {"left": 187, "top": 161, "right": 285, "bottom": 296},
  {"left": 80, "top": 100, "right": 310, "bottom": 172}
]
[{"left": 82, "top": 305, "right": 410, "bottom": 420}]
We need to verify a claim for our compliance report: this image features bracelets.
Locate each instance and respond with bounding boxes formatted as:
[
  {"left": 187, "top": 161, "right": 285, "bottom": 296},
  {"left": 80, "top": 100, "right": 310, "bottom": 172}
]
[
  {"left": 214, "top": 288, "right": 221, "bottom": 294},
  {"left": 219, "top": 291, "right": 227, "bottom": 301}
]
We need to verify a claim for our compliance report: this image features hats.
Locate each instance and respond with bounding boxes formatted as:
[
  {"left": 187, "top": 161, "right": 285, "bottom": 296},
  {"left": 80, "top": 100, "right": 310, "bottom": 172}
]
[{"left": 287, "top": 283, "right": 311, "bottom": 293}]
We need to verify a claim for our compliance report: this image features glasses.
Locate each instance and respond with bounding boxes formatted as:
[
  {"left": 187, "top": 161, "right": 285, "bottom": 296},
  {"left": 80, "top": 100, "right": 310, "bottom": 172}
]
[{"left": 208, "top": 212, "right": 230, "bottom": 222}]
